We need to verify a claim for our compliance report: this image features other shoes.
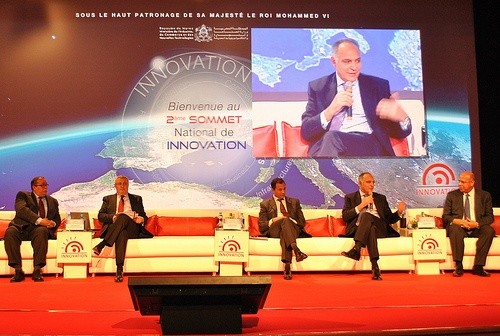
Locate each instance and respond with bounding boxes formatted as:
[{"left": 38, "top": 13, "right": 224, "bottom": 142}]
[
  {"left": 372, "top": 266, "right": 380, "bottom": 279},
  {"left": 92, "top": 247, "right": 102, "bottom": 255},
  {"left": 453, "top": 268, "right": 463, "bottom": 275},
  {"left": 342, "top": 248, "right": 360, "bottom": 260},
  {"left": 115, "top": 271, "right": 123, "bottom": 282},
  {"left": 32, "top": 269, "right": 43, "bottom": 281},
  {"left": 296, "top": 251, "right": 307, "bottom": 262},
  {"left": 473, "top": 266, "right": 490, "bottom": 276},
  {"left": 284, "top": 267, "right": 292, "bottom": 279},
  {"left": 11, "top": 274, "right": 25, "bottom": 282}
]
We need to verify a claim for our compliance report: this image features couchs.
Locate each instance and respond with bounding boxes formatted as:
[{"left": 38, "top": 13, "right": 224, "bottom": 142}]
[{"left": 0, "top": 208, "right": 500, "bottom": 275}]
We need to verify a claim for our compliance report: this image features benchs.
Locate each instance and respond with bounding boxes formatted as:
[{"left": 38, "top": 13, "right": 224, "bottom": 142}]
[{"left": 252, "top": 92, "right": 426, "bottom": 157}]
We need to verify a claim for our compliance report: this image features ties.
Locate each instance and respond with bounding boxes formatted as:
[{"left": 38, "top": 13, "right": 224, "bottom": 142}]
[
  {"left": 118, "top": 195, "right": 126, "bottom": 213},
  {"left": 277, "top": 198, "right": 286, "bottom": 216},
  {"left": 329, "top": 83, "right": 348, "bottom": 130},
  {"left": 38, "top": 197, "right": 45, "bottom": 219},
  {"left": 465, "top": 194, "right": 473, "bottom": 234},
  {"left": 355, "top": 195, "right": 369, "bottom": 226}
]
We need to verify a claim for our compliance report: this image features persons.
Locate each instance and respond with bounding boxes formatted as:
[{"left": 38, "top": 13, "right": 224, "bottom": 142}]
[
  {"left": 443, "top": 172, "right": 495, "bottom": 276},
  {"left": 341, "top": 172, "right": 406, "bottom": 281},
  {"left": 4, "top": 176, "right": 61, "bottom": 282},
  {"left": 301, "top": 38, "right": 412, "bottom": 158},
  {"left": 258, "top": 177, "right": 308, "bottom": 280},
  {"left": 93, "top": 176, "right": 148, "bottom": 283}
]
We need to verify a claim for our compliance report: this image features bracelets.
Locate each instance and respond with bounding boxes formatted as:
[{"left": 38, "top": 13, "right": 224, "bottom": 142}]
[{"left": 404, "top": 116, "right": 411, "bottom": 126}]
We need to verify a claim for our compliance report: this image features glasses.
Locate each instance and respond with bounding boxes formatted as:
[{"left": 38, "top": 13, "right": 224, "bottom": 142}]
[{"left": 34, "top": 184, "right": 49, "bottom": 188}]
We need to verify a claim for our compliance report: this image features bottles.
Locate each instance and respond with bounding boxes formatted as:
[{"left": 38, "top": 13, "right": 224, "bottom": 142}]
[
  {"left": 239, "top": 213, "right": 245, "bottom": 229},
  {"left": 228, "top": 213, "right": 233, "bottom": 219},
  {"left": 421, "top": 212, "right": 425, "bottom": 217},
  {"left": 400, "top": 214, "right": 406, "bottom": 228},
  {"left": 217, "top": 212, "right": 223, "bottom": 228},
  {"left": 80, "top": 214, "right": 86, "bottom": 231}
]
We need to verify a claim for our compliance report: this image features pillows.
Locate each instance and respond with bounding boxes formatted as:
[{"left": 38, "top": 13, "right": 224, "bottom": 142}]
[
  {"left": 252, "top": 121, "right": 280, "bottom": 158},
  {"left": 248, "top": 215, "right": 331, "bottom": 237},
  {"left": 157, "top": 216, "right": 218, "bottom": 236},
  {"left": 436, "top": 215, "right": 500, "bottom": 235},
  {"left": 330, "top": 215, "right": 348, "bottom": 237},
  {"left": 282, "top": 121, "right": 409, "bottom": 157},
  {"left": 92, "top": 215, "right": 156, "bottom": 238}
]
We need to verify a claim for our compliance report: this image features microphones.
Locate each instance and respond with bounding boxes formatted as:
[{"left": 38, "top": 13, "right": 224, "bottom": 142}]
[
  {"left": 369, "top": 191, "right": 373, "bottom": 210},
  {"left": 345, "top": 81, "right": 353, "bottom": 117}
]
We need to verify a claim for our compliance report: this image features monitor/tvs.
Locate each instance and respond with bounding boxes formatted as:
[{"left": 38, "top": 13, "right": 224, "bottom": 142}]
[{"left": 126, "top": 276, "right": 273, "bottom": 315}]
